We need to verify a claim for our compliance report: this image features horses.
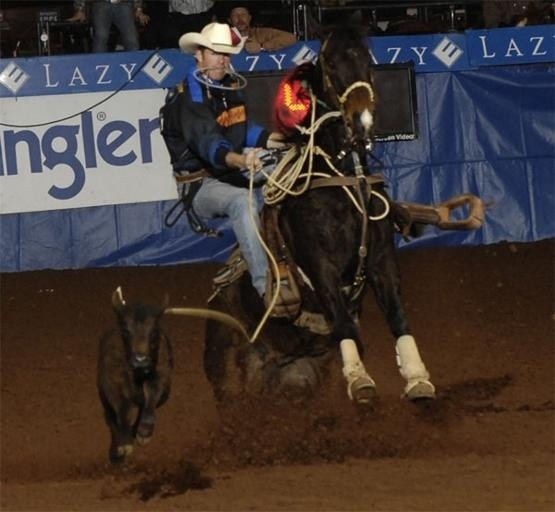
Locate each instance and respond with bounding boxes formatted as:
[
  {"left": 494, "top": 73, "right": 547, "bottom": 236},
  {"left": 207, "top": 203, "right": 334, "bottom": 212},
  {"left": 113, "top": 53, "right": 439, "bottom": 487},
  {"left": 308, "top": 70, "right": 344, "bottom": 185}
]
[{"left": 203, "top": 10, "right": 436, "bottom": 466}]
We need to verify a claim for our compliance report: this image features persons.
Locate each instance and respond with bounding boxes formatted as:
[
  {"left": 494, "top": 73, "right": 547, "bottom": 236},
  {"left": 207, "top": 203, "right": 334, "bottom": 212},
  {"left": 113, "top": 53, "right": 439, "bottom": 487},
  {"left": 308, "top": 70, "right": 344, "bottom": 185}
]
[
  {"left": 158, "top": 21, "right": 298, "bottom": 315},
  {"left": 266, "top": 0, "right": 555, "bottom": 41},
  {"left": 158, "top": 0, "right": 215, "bottom": 49},
  {"left": 66, "top": 0, "right": 152, "bottom": 53},
  {"left": 226, "top": 2, "right": 297, "bottom": 55}
]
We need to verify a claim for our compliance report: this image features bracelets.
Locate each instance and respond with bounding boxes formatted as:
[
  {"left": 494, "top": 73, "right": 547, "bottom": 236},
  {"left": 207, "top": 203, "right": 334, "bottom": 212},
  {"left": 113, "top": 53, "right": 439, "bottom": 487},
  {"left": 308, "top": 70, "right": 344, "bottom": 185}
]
[
  {"left": 260, "top": 43, "right": 264, "bottom": 52},
  {"left": 133, "top": 7, "right": 144, "bottom": 12}
]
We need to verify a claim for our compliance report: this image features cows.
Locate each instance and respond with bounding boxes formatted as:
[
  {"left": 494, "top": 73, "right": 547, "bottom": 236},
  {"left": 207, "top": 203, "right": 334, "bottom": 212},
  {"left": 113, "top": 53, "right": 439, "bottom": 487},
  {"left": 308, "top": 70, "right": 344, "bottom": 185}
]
[{"left": 97, "top": 286, "right": 174, "bottom": 483}]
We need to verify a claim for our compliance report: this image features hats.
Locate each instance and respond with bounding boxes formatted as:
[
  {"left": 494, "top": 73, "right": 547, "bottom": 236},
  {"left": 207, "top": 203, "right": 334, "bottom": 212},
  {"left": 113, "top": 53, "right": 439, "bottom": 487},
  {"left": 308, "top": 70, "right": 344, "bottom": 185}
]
[{"left": 177, "top": 19, "right": 251, "bottom": 56}]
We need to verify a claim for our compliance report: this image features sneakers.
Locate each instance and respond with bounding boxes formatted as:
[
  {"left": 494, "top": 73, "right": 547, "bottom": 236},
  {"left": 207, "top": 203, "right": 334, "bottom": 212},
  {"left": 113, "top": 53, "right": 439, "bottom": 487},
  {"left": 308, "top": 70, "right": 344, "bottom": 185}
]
[{"left": 262, "top": 283, "right": 299, "bottom": 312}]
